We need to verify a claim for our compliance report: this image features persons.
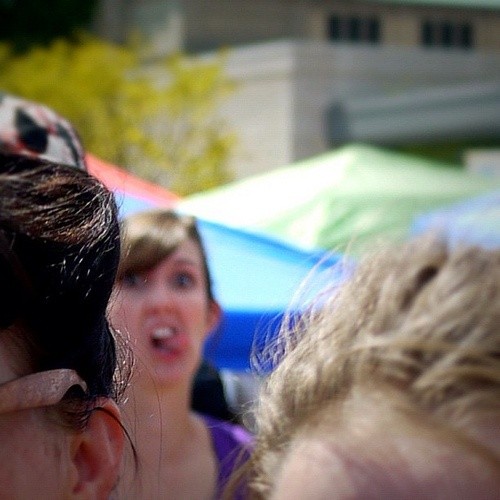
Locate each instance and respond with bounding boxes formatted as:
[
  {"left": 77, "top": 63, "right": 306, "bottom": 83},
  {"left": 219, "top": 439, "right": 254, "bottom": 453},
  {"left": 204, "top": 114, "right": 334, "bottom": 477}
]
[
  {"left": 219, "top": 226, "right": 499, "bottom": 499},
  {"left": 0, "top": 153, "right": 125, "bottom": 499},
  {"left": 105, "top": 212, "right": 256, "bottom": 499}
]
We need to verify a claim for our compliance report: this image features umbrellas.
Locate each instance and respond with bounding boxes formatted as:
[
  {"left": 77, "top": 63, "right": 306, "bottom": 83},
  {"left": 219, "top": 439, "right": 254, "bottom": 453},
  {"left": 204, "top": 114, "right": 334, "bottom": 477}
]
[{"left": 0, "top": 97, "right": 499, "bottom": 370}]
